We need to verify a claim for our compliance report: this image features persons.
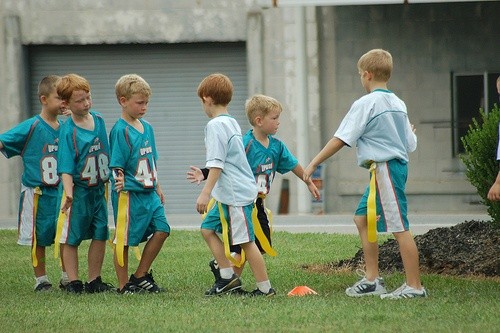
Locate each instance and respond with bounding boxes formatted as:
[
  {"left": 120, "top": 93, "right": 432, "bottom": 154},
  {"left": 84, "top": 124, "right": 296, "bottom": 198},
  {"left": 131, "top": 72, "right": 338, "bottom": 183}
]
[
  {"left": 187, "top": 93, "right": 322, "bottom": 294},
  {"left": 486, "top": 75, "right": 500, "bottom": 200},
  {"left": 0, "top": 70, "right": 171, "bottom": 297},
  {"left": 302, "top": 48, "right": 429, "bottom": 299},
  {"left": 195, "top": 70, "right": 276, "bottom": 297}
]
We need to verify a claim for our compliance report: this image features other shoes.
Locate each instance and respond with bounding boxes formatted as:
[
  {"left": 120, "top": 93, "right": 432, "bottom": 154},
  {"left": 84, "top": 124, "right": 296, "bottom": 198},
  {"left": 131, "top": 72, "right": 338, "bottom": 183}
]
[
  {"left": 58, "top": 279, "right": 73, "bottom": 292},
  {"left": 34, "top": 281, "right": 52, "bottom": 292}
]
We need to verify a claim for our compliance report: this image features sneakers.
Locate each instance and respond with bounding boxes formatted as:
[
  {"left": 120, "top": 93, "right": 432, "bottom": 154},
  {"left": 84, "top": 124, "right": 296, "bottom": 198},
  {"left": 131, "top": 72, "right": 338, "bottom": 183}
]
[
  {"left": 67, "top": 280, "right": 86, "bottom": 294},
  {"left": 117, "top": 283, "right": 151, "bottom": 295},
  {"left": 84, "top": 276, "right": 116, "bottom": 294},
  {"left": 345, "top": 268, "right": 386, "bottom": 297},
  {"left": 248, "top": 286, "right": 276, "bottom": 298},
  {"left": 209, "top": 259, "right": 221, "bottom": 280},
  {"left": 380, "top": 282, "right": 428, "bottom": 300},
  {"left": 204, "top": 274, "right": 243, "bottom": 296},
  {"left": 129, "top": 270, "right": 164, "bottom": 293}
]
